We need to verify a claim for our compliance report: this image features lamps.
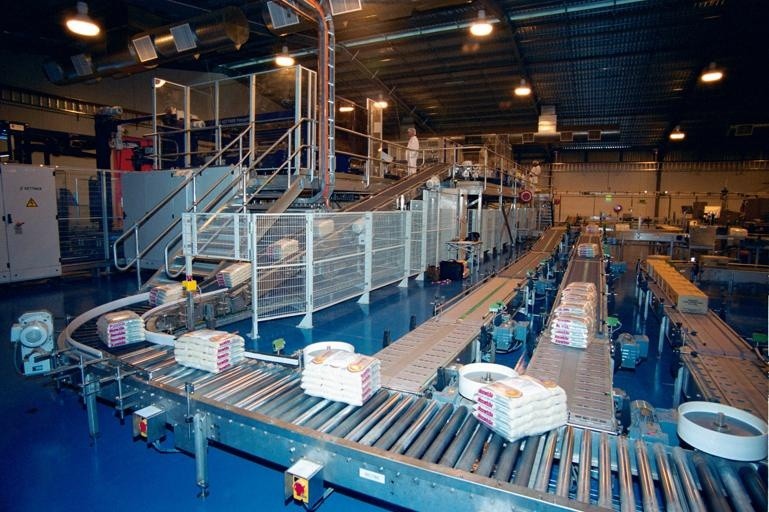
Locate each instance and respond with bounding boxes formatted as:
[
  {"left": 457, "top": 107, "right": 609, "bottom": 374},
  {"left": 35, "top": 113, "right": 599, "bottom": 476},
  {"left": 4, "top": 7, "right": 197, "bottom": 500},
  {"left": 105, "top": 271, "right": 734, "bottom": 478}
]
[
  {"left": 66, "top": 2, "right": 100, "bottom": 37},
  {"left": 670, "top": 126, "right": 684, "bottom": 140},
  {"left": 275, "top": 46, "right": 294, "bottom": 67},
  {"left": 700, "top": 63, "right": 723, "bottom": 82},
  {"left": 471, "top": 10, "right": 492, "bottom": 35},
  {"left": 514, "top": 77, "right": 530, "bottom": 96}
]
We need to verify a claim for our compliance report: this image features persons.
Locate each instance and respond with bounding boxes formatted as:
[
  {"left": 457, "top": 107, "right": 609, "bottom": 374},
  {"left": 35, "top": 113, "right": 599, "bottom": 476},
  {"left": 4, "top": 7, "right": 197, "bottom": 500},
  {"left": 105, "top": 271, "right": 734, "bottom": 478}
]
[
  {"left": 529, "top": 160, "right": 541, "bottom": 194},
  {"left": 405, "top": 127, "right": 419, "bottom": 176}
]
